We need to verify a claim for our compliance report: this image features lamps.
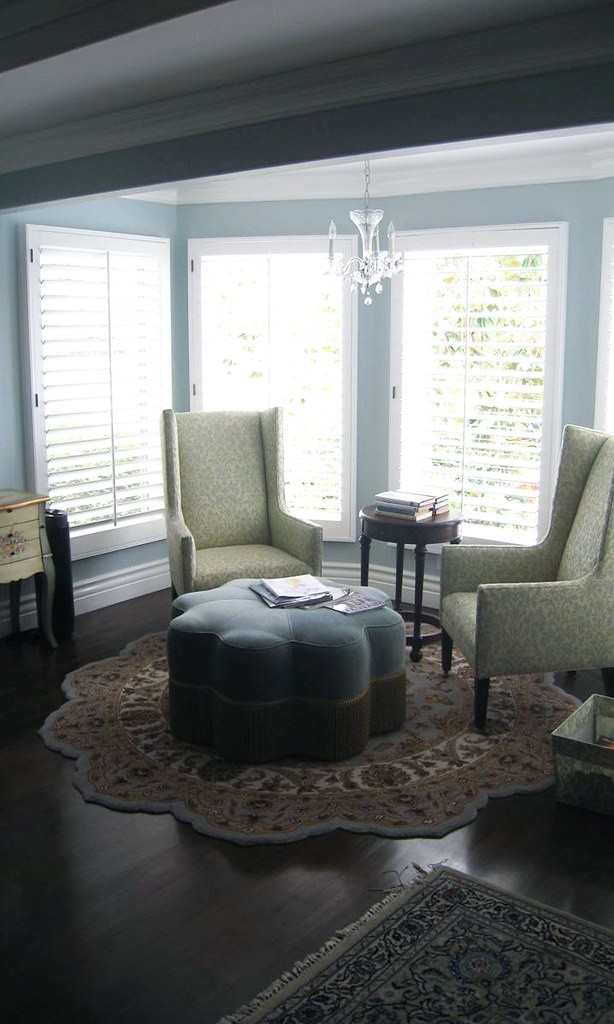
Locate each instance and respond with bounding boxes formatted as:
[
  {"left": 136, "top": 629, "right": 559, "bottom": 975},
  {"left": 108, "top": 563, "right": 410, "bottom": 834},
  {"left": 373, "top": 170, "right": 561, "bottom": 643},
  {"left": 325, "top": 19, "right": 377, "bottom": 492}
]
[{"left": 323, "top": 159, "right": 405, "bottom": 307}]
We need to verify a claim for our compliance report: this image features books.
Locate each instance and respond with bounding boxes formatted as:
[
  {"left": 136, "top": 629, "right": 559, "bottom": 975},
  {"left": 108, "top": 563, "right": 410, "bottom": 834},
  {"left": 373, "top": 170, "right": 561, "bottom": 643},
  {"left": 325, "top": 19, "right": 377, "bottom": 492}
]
[
  {"left": 248, "top": 574, "right": 388, "bottom": 616},
  {"left": 373, "top": 482, "right": 451, "bottom": 522}
]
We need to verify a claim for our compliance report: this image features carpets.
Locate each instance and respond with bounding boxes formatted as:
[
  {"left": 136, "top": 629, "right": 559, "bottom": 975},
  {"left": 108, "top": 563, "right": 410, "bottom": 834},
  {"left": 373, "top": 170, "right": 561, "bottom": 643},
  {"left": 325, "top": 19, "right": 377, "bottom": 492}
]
[
  {"left": 214, "top": 859, "right": 614, "bottom": 1024},
  {"left": 34, "top": 621, "right": 586, "bottom": 849}
]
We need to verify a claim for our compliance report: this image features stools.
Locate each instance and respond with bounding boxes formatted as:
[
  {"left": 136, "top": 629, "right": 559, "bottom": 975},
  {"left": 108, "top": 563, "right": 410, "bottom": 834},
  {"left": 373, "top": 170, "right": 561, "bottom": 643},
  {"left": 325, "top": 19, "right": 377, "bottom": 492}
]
[{"left": 164, "top": 576, "right": 407, "bottom": 763}]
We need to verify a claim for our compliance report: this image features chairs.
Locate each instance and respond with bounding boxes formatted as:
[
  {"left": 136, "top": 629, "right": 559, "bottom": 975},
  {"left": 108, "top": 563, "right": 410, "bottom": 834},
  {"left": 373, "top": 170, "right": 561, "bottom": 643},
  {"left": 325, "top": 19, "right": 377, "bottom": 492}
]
[
  {"left": 160, "top": 407, "right": 323, "bottom": 622},
  {"left": 440, "top": 423, "right": 614, "bottom": 735}
]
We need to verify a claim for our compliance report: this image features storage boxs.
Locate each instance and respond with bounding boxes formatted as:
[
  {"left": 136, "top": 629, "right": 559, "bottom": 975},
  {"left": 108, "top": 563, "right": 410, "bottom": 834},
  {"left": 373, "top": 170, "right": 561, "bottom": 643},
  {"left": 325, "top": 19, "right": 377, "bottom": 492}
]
[{"left": 551, "top": 694, "right": 614, "bottom": 817}]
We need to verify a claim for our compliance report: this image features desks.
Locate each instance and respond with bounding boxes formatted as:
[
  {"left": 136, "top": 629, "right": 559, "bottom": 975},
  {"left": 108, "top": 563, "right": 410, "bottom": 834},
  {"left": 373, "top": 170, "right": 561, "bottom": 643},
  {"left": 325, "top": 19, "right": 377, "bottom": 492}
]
[
  {"left": 0, "top": 487, "right": 60, "bottom": 651},
  {"left": 359, "top": 505, "right": 463, "bottom": 663}
]
